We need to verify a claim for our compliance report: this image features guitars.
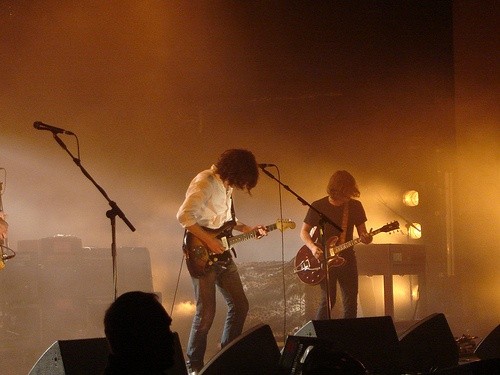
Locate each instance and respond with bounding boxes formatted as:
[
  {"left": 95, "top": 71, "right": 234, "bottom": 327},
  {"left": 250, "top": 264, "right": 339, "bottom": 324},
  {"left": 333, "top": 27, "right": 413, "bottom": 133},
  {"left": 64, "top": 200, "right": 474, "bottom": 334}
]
[
  {"left": 182, "top": 218, "right": 296, "bottom": 278},
  {"left": 294, "top": 221, "right": 400, "bottom": 285}
]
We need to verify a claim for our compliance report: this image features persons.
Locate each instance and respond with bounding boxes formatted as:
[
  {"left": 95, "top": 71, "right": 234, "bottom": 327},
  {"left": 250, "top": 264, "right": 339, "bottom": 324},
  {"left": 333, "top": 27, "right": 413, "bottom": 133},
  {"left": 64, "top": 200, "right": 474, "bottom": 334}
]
[
  {"left": 300, "top": 170, "right": 373, "bottom": 320},
  {"left": 103, "top": 291, "right": 179, "bottom": 375},
  {"left": 176, "top": 148, "right": 269, "bottom": 375},
  {"left": 0, "top": 170, "right": 8, "bottom": 270}
]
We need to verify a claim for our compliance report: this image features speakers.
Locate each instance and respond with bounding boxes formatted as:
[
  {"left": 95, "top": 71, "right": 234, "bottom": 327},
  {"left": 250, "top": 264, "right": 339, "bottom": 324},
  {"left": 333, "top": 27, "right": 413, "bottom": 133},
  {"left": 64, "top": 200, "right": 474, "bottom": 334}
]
[
  {"left": 473, "top": 324, "right": 500, "bottom": 360},
  {"left": 198, "top": 323, "right": 285, "bottom": 375},
  {"left": 27, "top": 331, "right": 189, "bottom": 375},
  {"left": 293, "top": 316, "right": 401, "bottom": 375},
  {"left": 399, "top": 313, "right": 459, "bottom": 375}
]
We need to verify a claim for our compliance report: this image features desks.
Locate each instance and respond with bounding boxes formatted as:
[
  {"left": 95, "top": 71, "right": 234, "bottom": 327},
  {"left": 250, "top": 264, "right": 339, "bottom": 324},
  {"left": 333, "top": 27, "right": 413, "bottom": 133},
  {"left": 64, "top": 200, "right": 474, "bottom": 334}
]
[{"left": 355, "top": 243, "right": 426, "bottom": 321}]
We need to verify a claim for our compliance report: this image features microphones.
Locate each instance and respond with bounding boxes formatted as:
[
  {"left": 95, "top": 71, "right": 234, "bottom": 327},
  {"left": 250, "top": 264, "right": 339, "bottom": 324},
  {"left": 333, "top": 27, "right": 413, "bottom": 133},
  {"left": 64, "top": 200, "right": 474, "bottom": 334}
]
[
  {"left": 257, "top": 163, "right": 274, "bottom": 167},
  {"left": 33, "top": 121, "right": 75, "bottom": 136}
]
